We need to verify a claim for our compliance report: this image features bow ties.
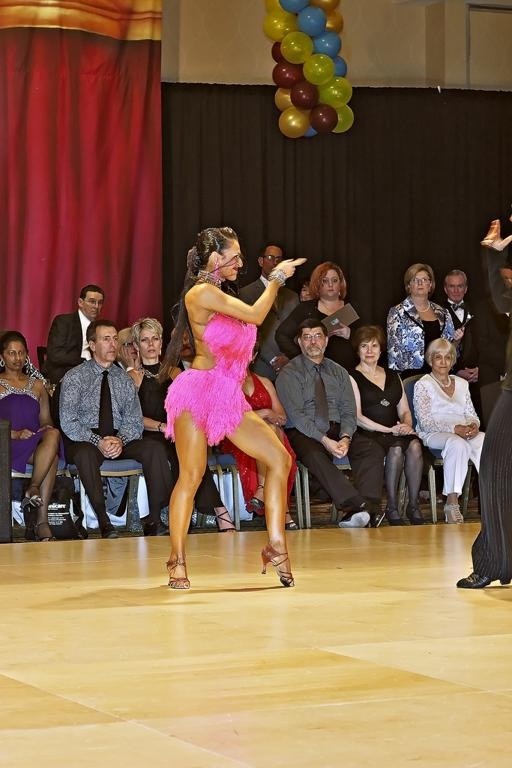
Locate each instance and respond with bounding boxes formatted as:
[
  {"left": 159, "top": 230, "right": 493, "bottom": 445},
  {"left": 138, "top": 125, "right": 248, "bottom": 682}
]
[{"left": 453, "top": 303, "right": 465, "bottom": 311}]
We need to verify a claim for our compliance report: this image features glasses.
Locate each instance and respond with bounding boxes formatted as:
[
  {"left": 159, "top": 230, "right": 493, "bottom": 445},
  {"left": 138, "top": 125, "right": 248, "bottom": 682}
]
[
  {"left": 123, "top": 342, "right": 133, "bottom": 347},
  {"left": 302, "top": 334, "right": 323, "bottom": 341},
  {"left": 262, "top": 255, "right": 281, "bottom": 261}
]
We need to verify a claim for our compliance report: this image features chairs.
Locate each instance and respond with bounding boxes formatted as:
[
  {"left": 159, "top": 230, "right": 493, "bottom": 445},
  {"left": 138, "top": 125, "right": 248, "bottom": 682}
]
[
  {"left": 297, "top": 457, "right": 351, "bottom": 527},
  {"left": 208, "top": 456, "right": 224, "bottom": 504},
  {"left": 68, "top": 460, "right": 143, "bottom": 531},
  {"left": 37, "top": 346, "right": 47, "bottom": 373},
  {"left": 219, "top": 455, "right": 303, "bottom": 530},
  {"left": 12, "top": 458, "right": 73, "bottom": 527},
  {"left": 401, "top": 375, "right": 472, "bottom": 523},
  {"left": 383, "top": 455, "right": 407, "bottom": 516}
]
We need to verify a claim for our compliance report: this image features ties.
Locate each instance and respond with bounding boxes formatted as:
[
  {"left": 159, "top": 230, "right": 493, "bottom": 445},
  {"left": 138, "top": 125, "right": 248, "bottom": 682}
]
[
  {"left": 314, "top": 365, "right": 330, "bottom": 432},
  {"left": 99, "top": 371, "right": 114, "bottom": 437}
]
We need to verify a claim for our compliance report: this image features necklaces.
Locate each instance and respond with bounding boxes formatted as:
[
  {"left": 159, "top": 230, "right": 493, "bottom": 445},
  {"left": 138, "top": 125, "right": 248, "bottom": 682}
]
[{"left": 195, "top": 269, "right": 223, "bottom": 290}]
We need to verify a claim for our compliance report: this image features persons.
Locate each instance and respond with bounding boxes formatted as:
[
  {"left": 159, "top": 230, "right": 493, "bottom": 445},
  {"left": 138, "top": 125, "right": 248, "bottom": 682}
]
[
  {"left": 234, "top": 243, "right": 488, "bottom": 527},
  {"left": 164, "top": 226, "right": 307, "bottom": 589},
  {"left": 453, "top": 217, "right": 510, "bottom": 587},
  {"left": 0, "top": 285, "right": 239, "bottom": 542}
]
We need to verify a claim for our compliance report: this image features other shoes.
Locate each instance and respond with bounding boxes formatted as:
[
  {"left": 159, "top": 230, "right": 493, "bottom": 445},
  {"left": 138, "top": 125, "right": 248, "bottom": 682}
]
[{"left": 20, "top": 495, "right": 464, "bottom": 542}]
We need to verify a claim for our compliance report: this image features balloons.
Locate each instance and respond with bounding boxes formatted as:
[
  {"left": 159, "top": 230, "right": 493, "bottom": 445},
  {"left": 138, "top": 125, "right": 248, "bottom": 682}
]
[{"left": 261, "top": 1, "right": 354, "bottom": 139}]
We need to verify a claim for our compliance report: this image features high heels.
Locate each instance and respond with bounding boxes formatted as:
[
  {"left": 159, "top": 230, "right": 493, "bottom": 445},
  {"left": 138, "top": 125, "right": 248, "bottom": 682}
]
[
  {"left": 457, "top": 572, "right": 510, "bottom": 588},
  {"left": 167, "top": 559, "right": 190, "bottom": 589},
  {"left": 262, "top": 543, "right": 294, "bottom": 586}
]
